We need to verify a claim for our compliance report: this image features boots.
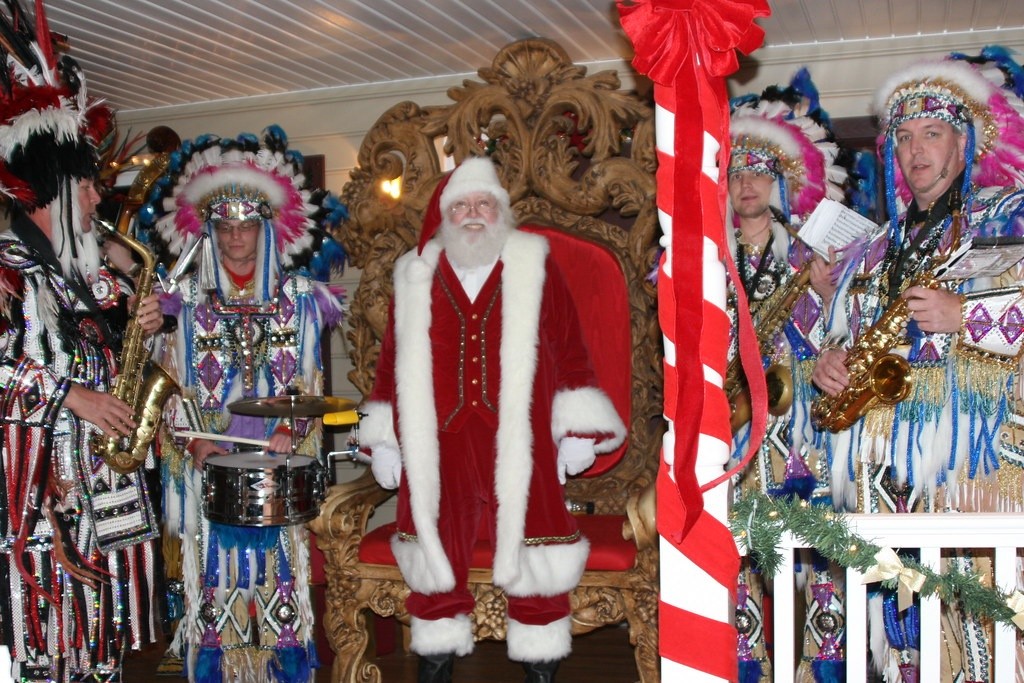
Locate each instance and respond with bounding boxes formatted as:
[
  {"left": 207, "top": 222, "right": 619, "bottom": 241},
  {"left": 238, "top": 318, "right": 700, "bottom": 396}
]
[
  {"left": 524, "top": 661, "right": 558, "bottom": 683},
  {"left": 417, "top": 653, "right": 453, "bottom": 683}
]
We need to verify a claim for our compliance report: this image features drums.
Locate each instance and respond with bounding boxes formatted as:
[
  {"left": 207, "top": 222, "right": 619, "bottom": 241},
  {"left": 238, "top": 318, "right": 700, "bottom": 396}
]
[{"left": 198, "top": 448, "right": 328, "bottom": 527}]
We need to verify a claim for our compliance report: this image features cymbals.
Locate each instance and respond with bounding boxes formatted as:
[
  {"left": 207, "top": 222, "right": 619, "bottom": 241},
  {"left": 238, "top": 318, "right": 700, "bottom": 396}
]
[{"left": 226, "top": 390, "right": 354, "bottom": 419}]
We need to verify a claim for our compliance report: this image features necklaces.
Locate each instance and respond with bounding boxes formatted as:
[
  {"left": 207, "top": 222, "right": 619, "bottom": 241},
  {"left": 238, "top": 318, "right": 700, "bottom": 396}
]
[
  {"left": 878, "top": 213, "right": 952, "bottom": 297},
  {"left": 733, "top": 244, "right": 779, "bottom": 355}
]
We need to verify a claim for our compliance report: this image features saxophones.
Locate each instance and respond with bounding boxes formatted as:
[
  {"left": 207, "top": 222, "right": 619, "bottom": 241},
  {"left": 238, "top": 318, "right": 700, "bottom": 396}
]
[
  {"left": 721, "top": 202, "right": 802, "bottom": 430},
  {"left": 85, "top": 215, "right": 178, "bottom": 475},
  {"left": 809, "top": 190, "right": 954, "bottom": 431}
]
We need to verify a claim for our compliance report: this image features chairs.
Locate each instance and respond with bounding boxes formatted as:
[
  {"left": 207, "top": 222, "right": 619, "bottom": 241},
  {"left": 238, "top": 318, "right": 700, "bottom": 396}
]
[{"left": 299, "top": 34, "right": 664, "bottom": 683}]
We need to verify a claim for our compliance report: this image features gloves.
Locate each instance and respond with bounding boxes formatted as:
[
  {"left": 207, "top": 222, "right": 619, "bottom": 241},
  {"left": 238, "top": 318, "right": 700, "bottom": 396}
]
[
  {"left": 370, "top": 444, "right": 402, "bottom": 489},
  {"left": 557, "top": 437, "right": 596, "bottom": 485}
]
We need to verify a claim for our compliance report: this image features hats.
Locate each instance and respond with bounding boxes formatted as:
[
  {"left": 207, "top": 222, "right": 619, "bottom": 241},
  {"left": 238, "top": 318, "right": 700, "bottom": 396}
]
[{"left": 406, "top": 155, "right": 510, "bottom": 282}]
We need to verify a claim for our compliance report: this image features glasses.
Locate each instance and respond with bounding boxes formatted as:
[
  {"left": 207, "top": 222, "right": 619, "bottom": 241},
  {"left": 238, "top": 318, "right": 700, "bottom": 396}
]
[{"left": 445, "top": 198, "right": 500, "bottom": 214}]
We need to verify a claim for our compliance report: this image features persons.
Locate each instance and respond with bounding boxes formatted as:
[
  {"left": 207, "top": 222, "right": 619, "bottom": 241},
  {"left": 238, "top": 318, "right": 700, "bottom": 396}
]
[
  {"left": 138, "top": 125, "right": 347, "bottom": 683},
  {"left": 808, "top": 45, "right": 1024, "bottom": 683},
  {"left": 725, "top": 67, "right": 850, "bottom": 683},
  {"left": 0, "top": 0, "right": 164, "bottom": 683},
  {"left": 356, "top": 157, "right": 625, "bottom": 683}
]
